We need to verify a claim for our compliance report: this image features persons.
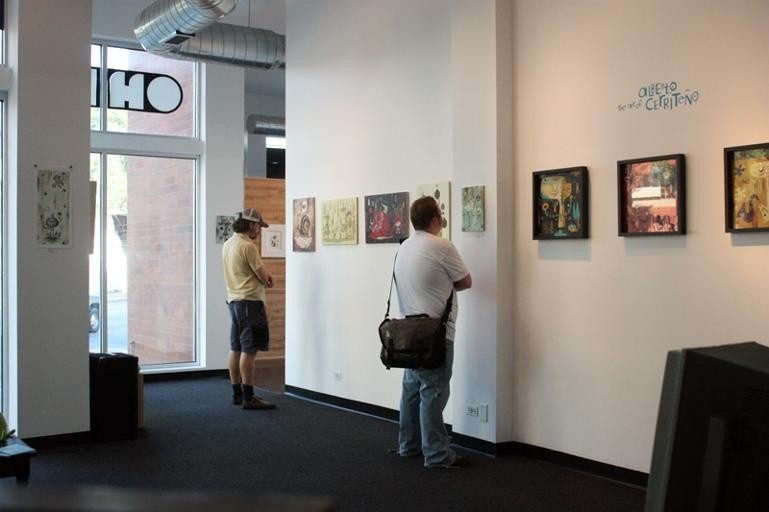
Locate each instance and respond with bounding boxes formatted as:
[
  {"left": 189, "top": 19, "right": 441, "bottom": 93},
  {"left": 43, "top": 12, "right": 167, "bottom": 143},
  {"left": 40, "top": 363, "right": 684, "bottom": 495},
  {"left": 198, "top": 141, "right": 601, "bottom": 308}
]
[
  {"left": 394, "top": 197, "right": 471, "bottom": 469},
  {"left": 222, "top": 209, "right": 276, "bottom": 410}
]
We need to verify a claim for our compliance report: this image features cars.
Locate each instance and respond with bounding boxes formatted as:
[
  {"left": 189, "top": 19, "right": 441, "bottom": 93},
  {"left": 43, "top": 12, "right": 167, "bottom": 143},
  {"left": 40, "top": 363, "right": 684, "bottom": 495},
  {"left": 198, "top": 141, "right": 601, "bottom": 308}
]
[{"left": 89, "top": 283, "right": 126, "bottom": 333}]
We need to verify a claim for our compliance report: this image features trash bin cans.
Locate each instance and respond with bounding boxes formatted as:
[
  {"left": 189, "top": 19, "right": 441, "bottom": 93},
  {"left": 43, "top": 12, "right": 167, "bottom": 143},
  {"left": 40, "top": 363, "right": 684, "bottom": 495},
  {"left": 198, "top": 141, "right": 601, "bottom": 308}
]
[{"left": 89, "top": 353, "right": 142, "bottom": 432}]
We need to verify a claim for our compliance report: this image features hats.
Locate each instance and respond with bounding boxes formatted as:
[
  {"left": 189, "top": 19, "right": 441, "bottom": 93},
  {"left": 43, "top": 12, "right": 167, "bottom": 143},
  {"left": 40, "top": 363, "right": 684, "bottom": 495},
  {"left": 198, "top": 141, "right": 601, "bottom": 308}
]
[{"left": 234, "top": 209, "right": 269, "bottom": 228}]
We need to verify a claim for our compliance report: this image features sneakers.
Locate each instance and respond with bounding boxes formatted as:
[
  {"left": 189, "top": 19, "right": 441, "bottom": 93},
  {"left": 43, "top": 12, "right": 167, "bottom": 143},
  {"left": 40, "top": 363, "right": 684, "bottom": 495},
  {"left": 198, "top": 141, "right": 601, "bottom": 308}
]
[
  {"left": 232, "top": 394, "right": 263, "bottom": 405},
  {"left": 426, "top": 455, "right": 482, "bottom": 470},
  {"left": 399, "top": 435, "right": 454, "bottom": 456},
  {"left": 242, "top": 396, "right": 277, "bottom": 410}
]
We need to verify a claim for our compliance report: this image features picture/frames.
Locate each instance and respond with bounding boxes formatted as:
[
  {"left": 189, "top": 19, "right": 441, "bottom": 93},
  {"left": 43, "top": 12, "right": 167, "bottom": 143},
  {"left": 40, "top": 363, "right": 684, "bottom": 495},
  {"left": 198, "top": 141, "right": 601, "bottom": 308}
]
[
  {"left": 616, "top": 154, "right": 687, "bottom": 237},
  {"left": 722, "top": 142, "right": 769, "bottom": 234},
  {"left": 532, "top": 165, "right": 589, "bottom": 240},
  {"left": 261, "top": 224, "right": 286, "bottom": 258}
]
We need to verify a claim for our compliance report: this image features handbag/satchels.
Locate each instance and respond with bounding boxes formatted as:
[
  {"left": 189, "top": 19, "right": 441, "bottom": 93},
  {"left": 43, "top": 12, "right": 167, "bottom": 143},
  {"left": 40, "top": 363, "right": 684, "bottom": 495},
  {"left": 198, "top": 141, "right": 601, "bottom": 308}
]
[{"left": 378, "top": 310, "right": 448, "bottom": 370}]
